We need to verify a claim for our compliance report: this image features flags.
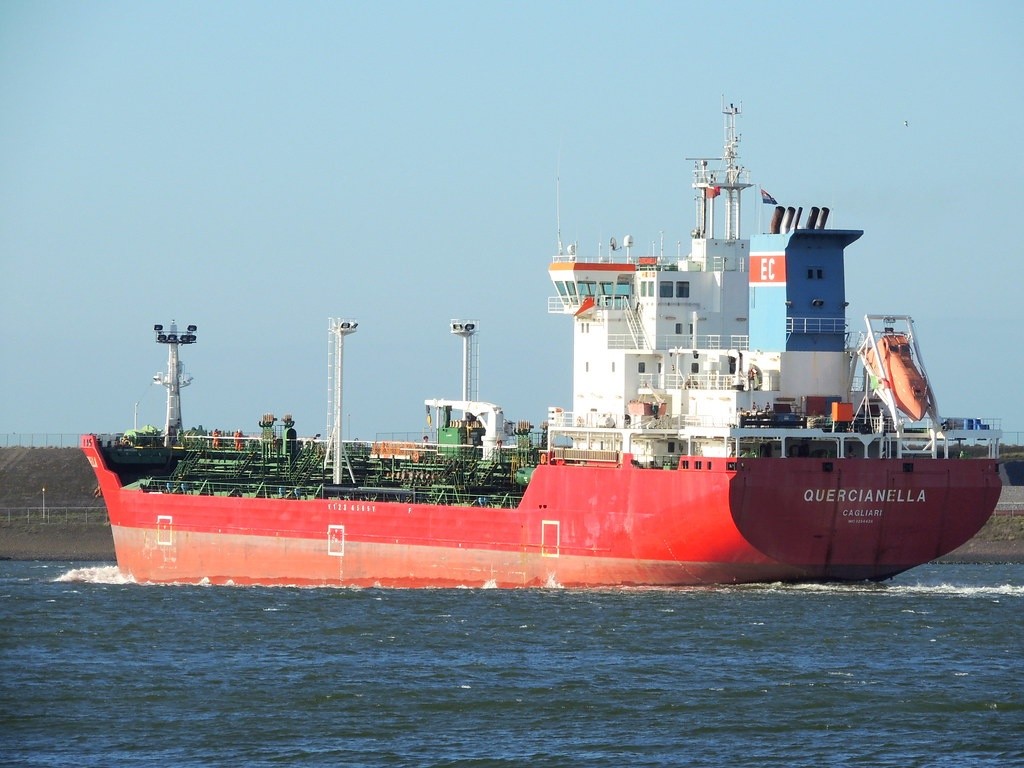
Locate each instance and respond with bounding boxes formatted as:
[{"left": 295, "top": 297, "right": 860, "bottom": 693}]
[{"left": 761, "top": 189, "right": 778, "bottom": 205}]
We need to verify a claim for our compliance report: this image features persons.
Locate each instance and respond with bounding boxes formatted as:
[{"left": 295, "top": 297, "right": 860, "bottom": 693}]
[
  {"left": 234, "top": 429, "right": 243, "bottom": 451},
  {"left": 212, "top": 429, "right": 219, "bottom": 451},
  {"left": 496, "top": 440, "right": 503, "bottom": 462},
  {"left": 422, "top": 436, "right": 431, "bottom": 464},
  {"left": 752, "top": 402, "right": 770, "bottom": 413},
  {"left": 596, "top": 414, "right": 616, "bottom": 428}
]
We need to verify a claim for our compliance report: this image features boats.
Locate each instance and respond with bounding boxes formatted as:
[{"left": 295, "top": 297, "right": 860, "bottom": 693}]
[{"left": 73, "top": 98, "right": 1007, "bottom": 589}]
